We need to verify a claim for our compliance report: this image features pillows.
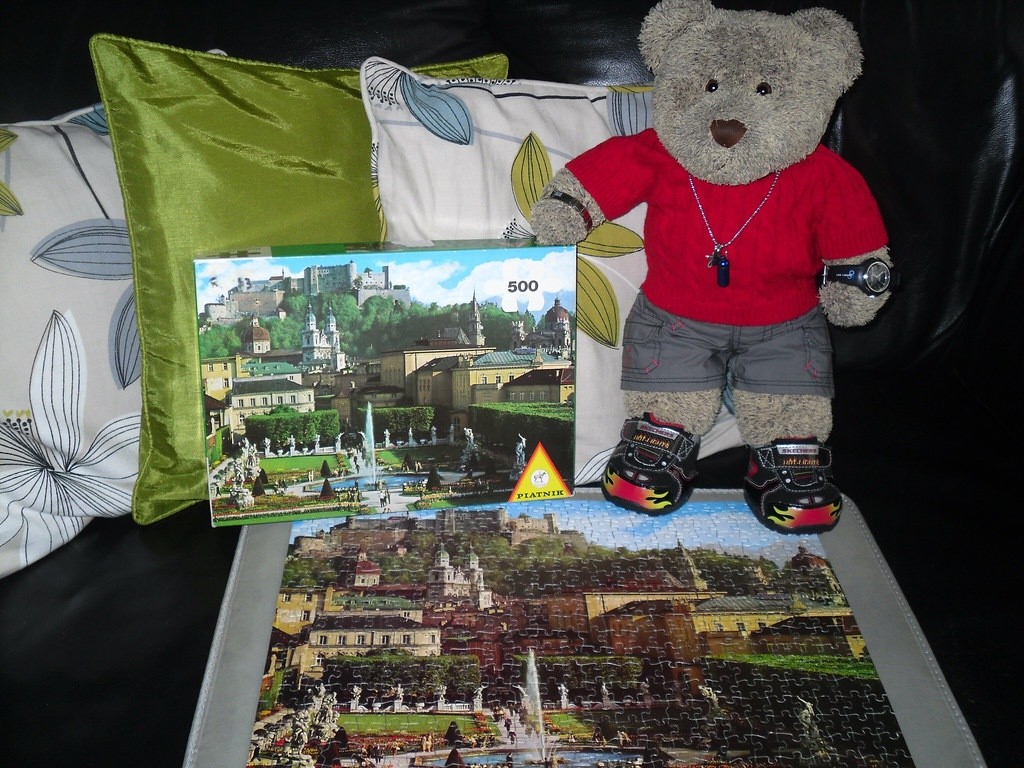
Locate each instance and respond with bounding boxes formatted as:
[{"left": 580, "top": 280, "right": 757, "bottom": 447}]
[{"left": 0, "top": 35, "right": 750, "bottom": 580}]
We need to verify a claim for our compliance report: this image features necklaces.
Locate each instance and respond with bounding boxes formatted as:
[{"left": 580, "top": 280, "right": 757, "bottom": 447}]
[{"left": 688, "top": 173, "right": 780, "bottom": 287}]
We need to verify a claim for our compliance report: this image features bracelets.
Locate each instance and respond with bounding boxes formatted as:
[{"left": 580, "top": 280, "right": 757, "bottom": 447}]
[{"left": 538, "top": 190, "right": 593, "bottom": 235}]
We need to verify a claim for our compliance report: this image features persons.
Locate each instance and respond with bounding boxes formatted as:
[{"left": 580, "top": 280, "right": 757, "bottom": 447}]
[
  {"left": 249, "top": 700, "right": 633, "bottom": 768},
  {"left": 402, "top": 478, "right": 427, "bottom": 491},
  {"left": 374, "top": 439, "right": 429, "bottom": 448},
  {"left": 379, "top": 490, "right": 391, "bottom": 508},
  {"left": 447, "top": 478, "right": 490, "bottom": 493},
  {"left": 344, "top": 442, "right": 364, "bottom": 474}
]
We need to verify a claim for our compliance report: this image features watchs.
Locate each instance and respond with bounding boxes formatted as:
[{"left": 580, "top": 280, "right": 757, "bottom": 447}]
[{"left": 815, "top": 258, "right": 892, "bottom": 299}]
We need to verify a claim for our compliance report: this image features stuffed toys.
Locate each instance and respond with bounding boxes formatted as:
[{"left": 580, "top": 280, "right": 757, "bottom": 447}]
[{"left": 532, "top": 0, "right": 894, "bottom": 534}]
